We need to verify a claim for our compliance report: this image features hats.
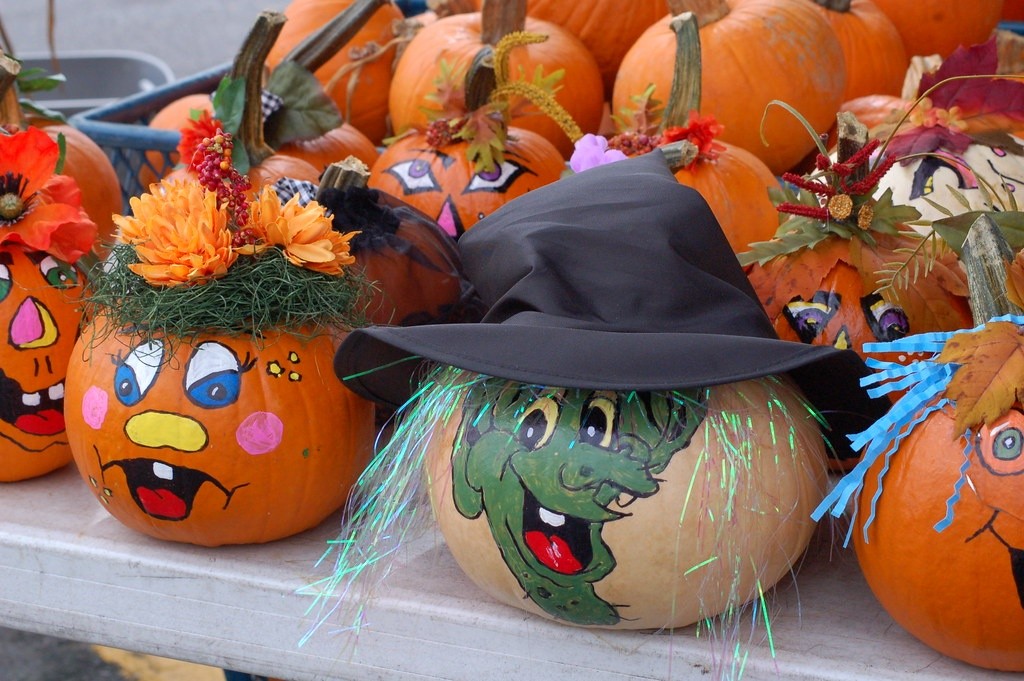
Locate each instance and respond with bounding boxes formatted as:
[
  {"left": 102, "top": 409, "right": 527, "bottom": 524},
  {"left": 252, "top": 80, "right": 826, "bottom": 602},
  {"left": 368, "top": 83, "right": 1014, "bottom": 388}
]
[{"left": 333, "top": 148, "right": 891, "bottom": 462}]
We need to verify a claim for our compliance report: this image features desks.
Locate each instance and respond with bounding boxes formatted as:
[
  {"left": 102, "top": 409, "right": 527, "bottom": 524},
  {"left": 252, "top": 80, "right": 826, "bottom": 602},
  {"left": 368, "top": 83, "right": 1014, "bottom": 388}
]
[{"left": 0, "top": 453, "right": 1024, "bottom": 681}]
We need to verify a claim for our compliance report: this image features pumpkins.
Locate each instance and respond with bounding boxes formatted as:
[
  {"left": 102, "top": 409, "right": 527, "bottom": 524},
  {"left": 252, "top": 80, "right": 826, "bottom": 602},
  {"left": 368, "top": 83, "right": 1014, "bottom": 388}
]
[{"left": 0, "top": 0, "right": 1024, "bottom": 675}]
